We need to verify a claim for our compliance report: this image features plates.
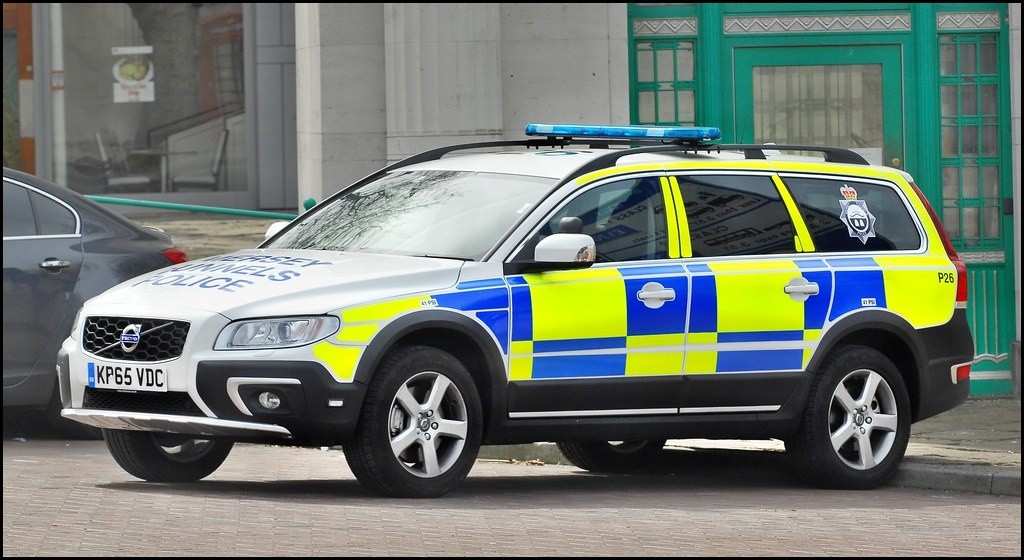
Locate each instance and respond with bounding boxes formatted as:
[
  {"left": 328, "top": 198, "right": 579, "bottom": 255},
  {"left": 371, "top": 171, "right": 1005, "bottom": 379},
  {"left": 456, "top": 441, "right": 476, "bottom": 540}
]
[{"left": 113, "top": 58, "right": 153, "bottom": 84}]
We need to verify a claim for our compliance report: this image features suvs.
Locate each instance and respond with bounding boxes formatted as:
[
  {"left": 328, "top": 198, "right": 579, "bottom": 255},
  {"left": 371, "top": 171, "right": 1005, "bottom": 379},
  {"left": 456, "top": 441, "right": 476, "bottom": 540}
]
[{"left": 53, "top": 123, "right": 976, "bottom": 501}]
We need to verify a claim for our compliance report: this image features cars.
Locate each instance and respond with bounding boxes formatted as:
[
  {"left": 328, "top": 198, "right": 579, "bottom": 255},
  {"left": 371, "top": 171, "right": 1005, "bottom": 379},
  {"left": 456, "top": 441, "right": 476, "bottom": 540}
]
[{"left": 1, "top": 166, "right": 193, "bottom": 412}]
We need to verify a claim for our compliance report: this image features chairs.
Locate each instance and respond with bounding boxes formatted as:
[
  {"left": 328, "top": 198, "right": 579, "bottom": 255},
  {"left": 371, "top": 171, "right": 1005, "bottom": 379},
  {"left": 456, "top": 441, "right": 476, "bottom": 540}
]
[
  {"left": 599, "top": 194, "right": 655, "bottom": 262},
  {"left": 170, "top": 129, "right": 230, "bottom": 191},
  {"left": 94, "top": 128, "right": 159, "bottom": 191}
]
